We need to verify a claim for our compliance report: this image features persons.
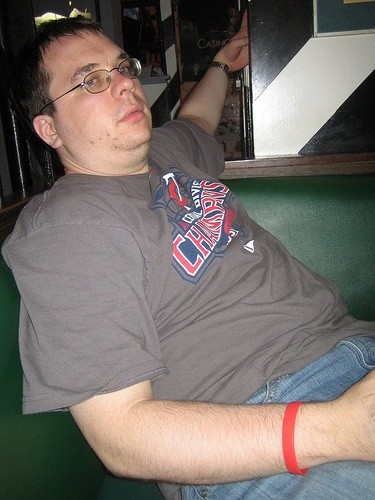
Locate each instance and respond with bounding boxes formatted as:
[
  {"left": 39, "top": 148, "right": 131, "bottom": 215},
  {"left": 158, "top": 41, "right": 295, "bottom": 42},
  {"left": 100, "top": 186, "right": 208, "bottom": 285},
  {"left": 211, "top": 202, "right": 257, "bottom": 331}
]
[{"left": 0, "top": 9, "right": 375, "bottom": 500}]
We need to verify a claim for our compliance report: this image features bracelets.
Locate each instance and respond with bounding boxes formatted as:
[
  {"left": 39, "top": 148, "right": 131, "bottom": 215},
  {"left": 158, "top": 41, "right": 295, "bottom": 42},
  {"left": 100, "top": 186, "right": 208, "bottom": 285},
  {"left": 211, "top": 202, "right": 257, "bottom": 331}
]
[
  {"left": 281, "top": 402, "right": 308, "bottom": 475},
  {"left": 210, "top": 61, "right": 231, "bottom": 79}
]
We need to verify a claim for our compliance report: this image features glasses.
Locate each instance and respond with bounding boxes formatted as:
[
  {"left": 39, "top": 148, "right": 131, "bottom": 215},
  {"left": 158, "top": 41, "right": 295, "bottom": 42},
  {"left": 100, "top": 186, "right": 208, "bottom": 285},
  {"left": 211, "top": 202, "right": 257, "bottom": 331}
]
[{"left": 39, "top": 56, "right": 143, "bottom": 117}]
